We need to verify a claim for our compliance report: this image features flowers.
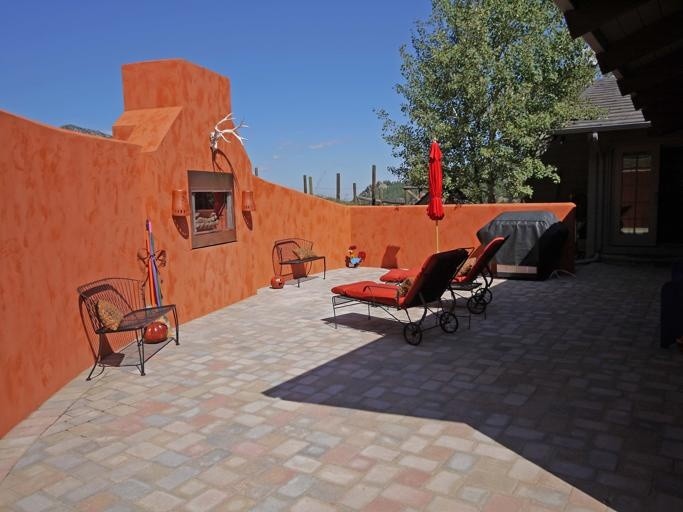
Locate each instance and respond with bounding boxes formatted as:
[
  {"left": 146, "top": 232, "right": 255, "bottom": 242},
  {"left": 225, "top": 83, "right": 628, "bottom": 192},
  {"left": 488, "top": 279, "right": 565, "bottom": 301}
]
[{"left": 345, "top": 245, "right": 366, "bottom": 264}]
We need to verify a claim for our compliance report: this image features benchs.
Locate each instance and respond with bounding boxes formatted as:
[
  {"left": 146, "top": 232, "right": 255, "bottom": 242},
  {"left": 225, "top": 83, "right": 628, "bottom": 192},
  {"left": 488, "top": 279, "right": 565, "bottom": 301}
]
[
  {"left": 77, "top": 276, "right": 179, "bottom": 381},
  {"left": 275, "top": 237, "right": 326, "bottom": 285}
]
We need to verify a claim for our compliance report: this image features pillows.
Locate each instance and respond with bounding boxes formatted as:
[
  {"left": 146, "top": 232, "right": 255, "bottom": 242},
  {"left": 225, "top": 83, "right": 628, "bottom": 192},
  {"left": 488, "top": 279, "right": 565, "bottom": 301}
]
[
  {"left": 91, "top": 299, "right": 123, "bottom": 331},
  {"left": 293, "top": 247, "right": 317, "bottom": 261},
  {"left": 395, "top": 275, "right": 416, "bottom": 298},
  {"left": 455, "top": 257, "right": 477, "bottom": 277}
]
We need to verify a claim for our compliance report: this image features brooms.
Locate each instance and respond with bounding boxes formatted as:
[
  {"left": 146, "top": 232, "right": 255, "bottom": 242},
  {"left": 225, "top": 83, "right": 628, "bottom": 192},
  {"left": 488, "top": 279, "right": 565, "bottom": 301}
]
[{"left": 145, "top": 217, "right": 174, "bottom": 340}]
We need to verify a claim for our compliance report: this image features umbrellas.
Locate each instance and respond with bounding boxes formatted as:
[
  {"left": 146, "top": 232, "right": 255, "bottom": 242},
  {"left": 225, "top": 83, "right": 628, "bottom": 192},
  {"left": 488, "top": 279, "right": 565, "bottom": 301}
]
[{"left": 427, "top": 139, "right": 444, "bottom": 254}]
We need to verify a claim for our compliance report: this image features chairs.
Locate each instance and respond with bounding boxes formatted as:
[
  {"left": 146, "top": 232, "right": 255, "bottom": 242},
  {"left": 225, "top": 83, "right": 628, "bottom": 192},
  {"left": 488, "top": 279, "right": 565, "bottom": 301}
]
[
  {"left": 331, "top": 247, "right": 475, "bottom": 345},
  {"left": 379, "top": 234, "right": 511, "bottom": 314}
]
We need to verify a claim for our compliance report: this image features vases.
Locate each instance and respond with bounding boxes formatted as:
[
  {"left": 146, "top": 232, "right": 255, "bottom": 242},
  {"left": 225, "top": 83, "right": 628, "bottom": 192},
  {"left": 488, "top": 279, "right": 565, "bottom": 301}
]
[{"left": 347, "top": 261, "right": 357, "bottom": 268}]
